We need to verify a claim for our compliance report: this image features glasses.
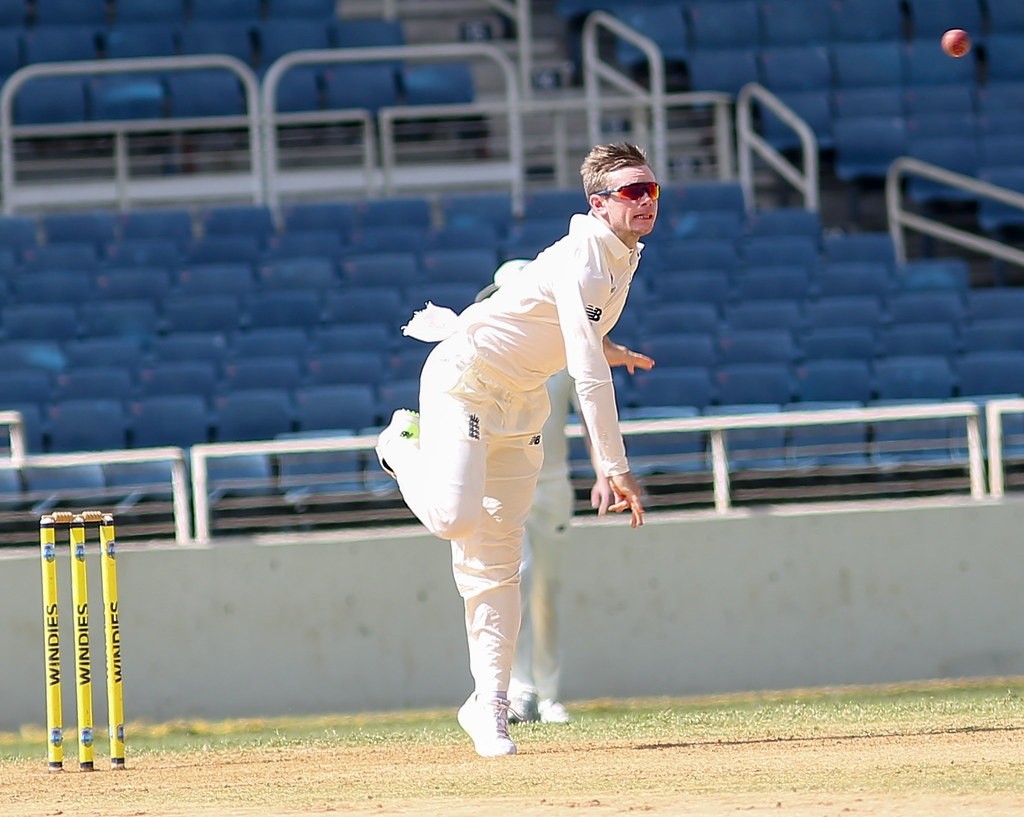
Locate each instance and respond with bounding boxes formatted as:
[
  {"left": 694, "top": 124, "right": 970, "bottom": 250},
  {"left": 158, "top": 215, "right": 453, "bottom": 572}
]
[{"left": 595, "top": 181, "right": 660, "bottom": 200}]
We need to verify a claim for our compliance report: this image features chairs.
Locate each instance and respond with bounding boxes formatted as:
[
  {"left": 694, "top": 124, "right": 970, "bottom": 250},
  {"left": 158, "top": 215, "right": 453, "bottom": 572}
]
[{"left": 0, "top": 0, "right": 1024, "bottom": 501}]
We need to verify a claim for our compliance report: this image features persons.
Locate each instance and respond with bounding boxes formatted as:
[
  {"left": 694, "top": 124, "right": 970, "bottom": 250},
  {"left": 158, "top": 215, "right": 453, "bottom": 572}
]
[
  {"left": 496, "top": 258, "right": 611, "bottom": 724},
  {"left": 374, "top": 144, "right": 659, "bottom": 757}
]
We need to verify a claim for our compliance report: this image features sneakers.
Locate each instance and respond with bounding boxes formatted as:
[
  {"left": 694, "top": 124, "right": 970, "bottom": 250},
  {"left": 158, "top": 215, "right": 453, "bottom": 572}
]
[
  {"left": 514, "top": 690, "right": 564, "bottom": 720},
  {"left": 457, "top": 698, "right": 515, "bottom": 756},
  {"left": 371, "top": 408, "right": 415, "bottom": 467}
]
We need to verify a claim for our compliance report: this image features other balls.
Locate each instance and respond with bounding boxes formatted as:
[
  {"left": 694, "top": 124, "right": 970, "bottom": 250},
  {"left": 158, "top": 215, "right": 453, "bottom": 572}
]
[{"left": 941, "top": 29, "right": 972, "bottom": 58}]
